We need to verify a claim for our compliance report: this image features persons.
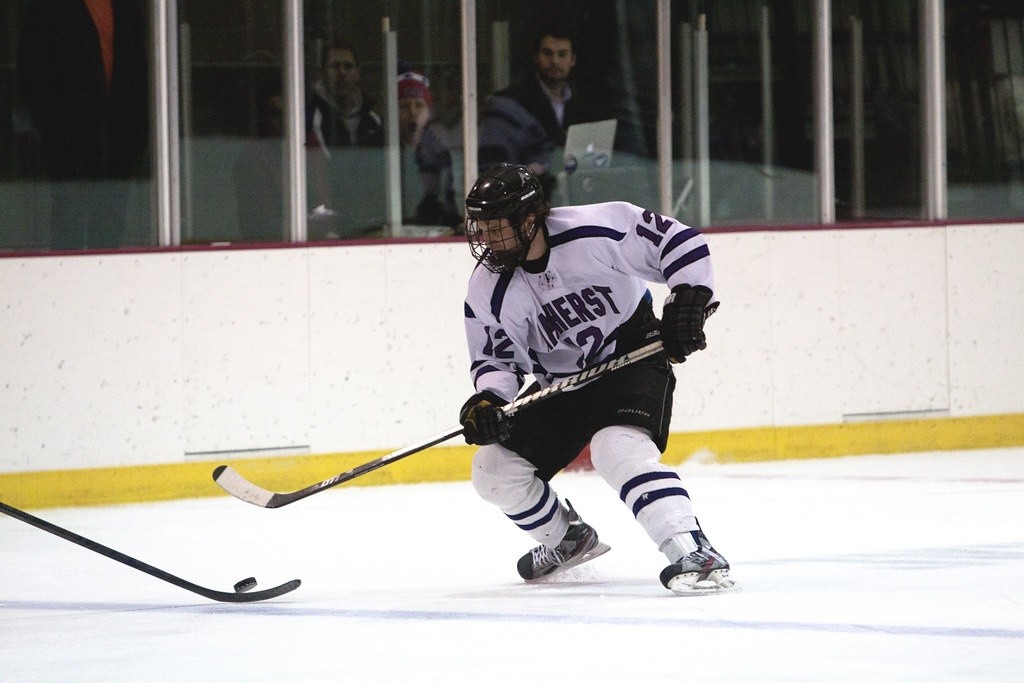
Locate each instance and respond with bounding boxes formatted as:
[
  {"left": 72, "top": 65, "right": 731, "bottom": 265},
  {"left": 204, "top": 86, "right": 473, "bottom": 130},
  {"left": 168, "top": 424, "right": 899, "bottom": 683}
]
[
  {"left": 14, "top": 0, "right": 152, "bottom": 252},
  {"left": 481, "top": 26, "right": 603, "bottom": 211},
  {"left": 230, "top": 40, "right": 460, "bottom": 244},
  {"left": 461, "top": 161, "right": 730, "bottom": 592}
]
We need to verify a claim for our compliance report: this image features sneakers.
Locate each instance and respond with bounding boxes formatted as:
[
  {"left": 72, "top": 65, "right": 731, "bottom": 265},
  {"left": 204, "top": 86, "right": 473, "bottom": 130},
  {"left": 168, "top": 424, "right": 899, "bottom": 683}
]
[
  {"left": 660, "top": 516, "right": 735, "bottom": 596},
  {"left": 517, "top": 498, "right": 611, "bottom": 582}
]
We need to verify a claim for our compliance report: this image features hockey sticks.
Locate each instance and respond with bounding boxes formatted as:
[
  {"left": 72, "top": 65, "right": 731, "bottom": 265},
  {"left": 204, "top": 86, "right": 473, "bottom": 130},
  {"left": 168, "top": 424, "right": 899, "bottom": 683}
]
[
  {"left": 210, "top": 338, "right": 664, "bottom": 509},
  {"left": 1, "top": 501, "right": 301, "bottom": 604}
]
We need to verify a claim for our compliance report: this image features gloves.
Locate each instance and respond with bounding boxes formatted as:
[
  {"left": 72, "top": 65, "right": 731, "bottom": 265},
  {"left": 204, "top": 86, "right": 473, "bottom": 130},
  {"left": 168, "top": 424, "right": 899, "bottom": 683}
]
[
  {"left": 657, "top": 289, "right": 706, "bottom": 363},
  {"left": 459, "top": 390, "right": 516, "bottom": 445}
]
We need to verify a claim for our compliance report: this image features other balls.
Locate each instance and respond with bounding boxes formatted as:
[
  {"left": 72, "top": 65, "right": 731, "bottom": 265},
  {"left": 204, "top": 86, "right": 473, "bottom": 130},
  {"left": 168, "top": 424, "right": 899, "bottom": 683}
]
[{"left": 233, "top": 577, "right": 258, "bottom": 593}]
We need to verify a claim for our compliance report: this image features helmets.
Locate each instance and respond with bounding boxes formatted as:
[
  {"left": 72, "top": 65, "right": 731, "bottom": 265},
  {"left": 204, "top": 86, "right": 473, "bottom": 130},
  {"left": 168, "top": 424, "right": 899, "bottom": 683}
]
[{"left": 465, "top": 162, "right": 548, "bottom": 268}]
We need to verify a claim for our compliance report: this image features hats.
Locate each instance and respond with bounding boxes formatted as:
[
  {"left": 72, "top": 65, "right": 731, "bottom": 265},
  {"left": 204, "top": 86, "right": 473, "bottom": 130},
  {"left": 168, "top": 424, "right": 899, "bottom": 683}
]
[{"left": 396, "top": 71, "right": 433, "bottom": 108}]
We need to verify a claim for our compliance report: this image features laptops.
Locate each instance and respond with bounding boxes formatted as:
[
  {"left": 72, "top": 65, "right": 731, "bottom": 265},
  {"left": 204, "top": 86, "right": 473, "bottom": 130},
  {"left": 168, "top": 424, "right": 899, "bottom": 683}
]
[{"left": 564, "top": 119, "right": 617, "bottom": 170}]
